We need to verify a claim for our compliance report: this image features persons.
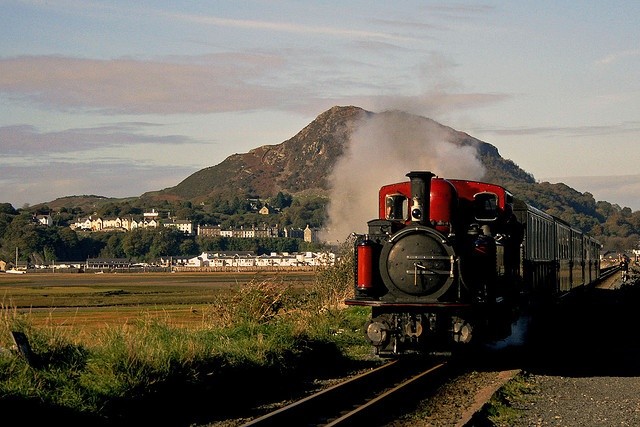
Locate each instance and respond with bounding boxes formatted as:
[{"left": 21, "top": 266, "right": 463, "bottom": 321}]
[
  {"left": 619, "top": 258, "right": 628, "bottom": 282},
  {"left": 623, "top": 253, "right": 629, "bottom": 267}
]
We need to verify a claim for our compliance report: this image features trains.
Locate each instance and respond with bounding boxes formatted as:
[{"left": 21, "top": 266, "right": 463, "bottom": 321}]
[{"left": 343, "top": 171, "right": 603, "bottom": 357}]
